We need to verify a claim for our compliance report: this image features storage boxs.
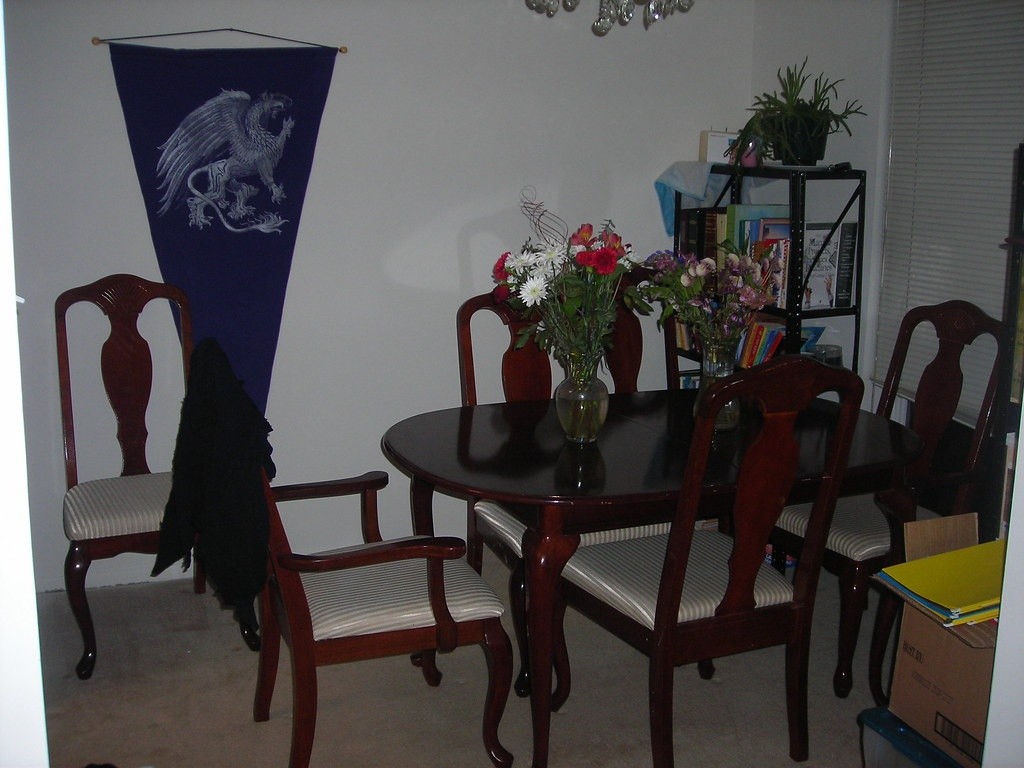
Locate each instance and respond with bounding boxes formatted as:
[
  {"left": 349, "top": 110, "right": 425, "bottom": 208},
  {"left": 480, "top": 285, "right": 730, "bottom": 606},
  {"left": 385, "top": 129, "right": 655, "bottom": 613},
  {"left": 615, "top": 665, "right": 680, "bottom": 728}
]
[{"left": 868, "top": 513, "right": 1024, "bottom": 768}]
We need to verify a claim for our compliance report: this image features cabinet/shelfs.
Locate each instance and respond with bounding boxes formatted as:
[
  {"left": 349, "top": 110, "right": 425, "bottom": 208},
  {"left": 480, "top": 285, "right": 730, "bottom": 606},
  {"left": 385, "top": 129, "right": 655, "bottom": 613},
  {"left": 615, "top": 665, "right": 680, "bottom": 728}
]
[{"left": 665, "top": 166, "right": 867, "bottom": 395}]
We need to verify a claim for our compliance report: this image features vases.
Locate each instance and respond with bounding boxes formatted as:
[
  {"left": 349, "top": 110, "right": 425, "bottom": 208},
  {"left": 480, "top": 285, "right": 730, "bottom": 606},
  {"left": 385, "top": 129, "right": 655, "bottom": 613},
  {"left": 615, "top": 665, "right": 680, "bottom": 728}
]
[
  {"left": 693, "top": 338, "right": 739, "bottom": 431},
  {"left": 555, "top": 355, "right": 609, "bottom": 443}
]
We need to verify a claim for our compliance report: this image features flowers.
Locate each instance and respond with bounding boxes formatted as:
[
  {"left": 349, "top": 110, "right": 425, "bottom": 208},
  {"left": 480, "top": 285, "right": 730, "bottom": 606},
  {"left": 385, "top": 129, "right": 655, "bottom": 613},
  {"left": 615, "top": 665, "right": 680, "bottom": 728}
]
[
  {"left": 641, "top": 239, "right": 782, "bottom": 353},
  {"left": 493, "top": 204, "right": 635, "bottom": 431}
]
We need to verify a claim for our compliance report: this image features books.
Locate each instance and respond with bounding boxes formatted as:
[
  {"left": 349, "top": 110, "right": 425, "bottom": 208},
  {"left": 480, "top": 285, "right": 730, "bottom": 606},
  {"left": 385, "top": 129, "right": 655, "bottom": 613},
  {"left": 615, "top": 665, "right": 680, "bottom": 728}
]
[
  {"left": 679, "top": 203, "right": 858, "bottom": 309},
  {"left": 675, "top": 318, "right": 826, "bottom": 368}
]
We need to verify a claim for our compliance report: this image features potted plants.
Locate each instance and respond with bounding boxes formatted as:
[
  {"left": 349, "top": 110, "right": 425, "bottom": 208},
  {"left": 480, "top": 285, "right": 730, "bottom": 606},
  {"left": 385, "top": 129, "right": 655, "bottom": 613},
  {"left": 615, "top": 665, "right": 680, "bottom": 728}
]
[{"left": 714, "top": 56, "right": 867, "bottom": 208}]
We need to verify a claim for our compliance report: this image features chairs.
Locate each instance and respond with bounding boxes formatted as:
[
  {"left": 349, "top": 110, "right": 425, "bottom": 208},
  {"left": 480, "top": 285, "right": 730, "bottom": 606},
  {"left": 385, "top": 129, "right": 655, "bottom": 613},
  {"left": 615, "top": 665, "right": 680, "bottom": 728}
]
[
  {"left": 766, "top": 298, "right": 1005, "bottom": 697},
  {"left": 456, "top": 294, "right": 642, "bottom": 698},
  {"left": 552, "top": 356, "right": 863, "bottom": 768},
  {"left": 196, "top": 349, "right": 514, "bottom": 767},
  {"left": 53, "top": 275, "right": 260, "bottom": 682}
]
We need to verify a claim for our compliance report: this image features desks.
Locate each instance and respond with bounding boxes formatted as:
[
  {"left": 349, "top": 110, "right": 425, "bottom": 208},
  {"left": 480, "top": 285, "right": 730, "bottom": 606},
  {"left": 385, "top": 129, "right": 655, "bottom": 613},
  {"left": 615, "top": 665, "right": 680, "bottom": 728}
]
[{"left": 379, "top": 390, "right": 925, "bottom": 768}]
